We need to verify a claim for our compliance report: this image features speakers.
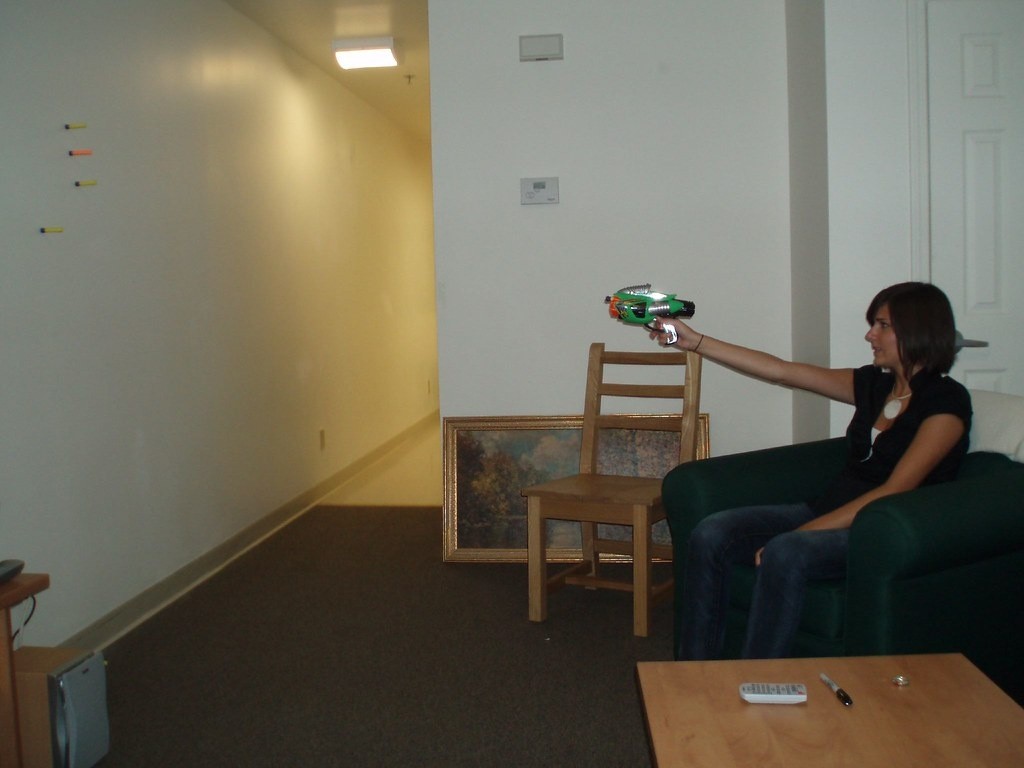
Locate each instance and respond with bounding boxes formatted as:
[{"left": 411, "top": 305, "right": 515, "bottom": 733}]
[{"left": 12, "top": 647, "right": 110, "bottom": 768}]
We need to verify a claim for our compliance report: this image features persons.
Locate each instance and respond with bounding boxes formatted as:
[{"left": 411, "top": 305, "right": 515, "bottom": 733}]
[{"left": 648, "top": 283, "right": 972, "bottom": 660}]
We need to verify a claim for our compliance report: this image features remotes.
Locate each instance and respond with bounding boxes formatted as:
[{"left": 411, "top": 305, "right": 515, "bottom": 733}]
[{"left": 740, "top": 683, "right": 808, "bottom": 703}]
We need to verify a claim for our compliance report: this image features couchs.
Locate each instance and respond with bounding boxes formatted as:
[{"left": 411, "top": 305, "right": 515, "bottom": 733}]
[{"left": 659, "top": 436, "right": 1024, "bottom": 662}]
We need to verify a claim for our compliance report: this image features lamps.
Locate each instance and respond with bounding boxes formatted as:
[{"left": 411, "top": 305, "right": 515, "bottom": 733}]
[{"left": 330, "top": 35, "right": 399, "bottom": 70}]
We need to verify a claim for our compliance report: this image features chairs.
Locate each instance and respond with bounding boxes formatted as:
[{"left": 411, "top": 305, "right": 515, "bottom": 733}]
[{"left": 520, "top": 341, "right": 704, "bottom": 639}]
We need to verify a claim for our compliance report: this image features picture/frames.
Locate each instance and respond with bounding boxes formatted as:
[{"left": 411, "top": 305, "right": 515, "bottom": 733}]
[{"left": 442, "top": 413, "right": 709, "bottom": 564}]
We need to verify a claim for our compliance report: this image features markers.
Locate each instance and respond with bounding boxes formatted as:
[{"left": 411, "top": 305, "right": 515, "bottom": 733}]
[{"left": 818, "top": 672, "right": 853, "bottom": 707}]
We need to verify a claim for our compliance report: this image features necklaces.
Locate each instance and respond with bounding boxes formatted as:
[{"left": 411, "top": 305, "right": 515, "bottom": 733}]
[{"left": 883, "top": 384, "right": 911, "bottom": 420}]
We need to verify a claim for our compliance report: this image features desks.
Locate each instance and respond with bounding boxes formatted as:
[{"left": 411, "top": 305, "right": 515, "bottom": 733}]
[
  {"left": 0, "top": 572, "right": 54, "bottom": 767},
  {"left": 636, "top": 653, "right": 1024, "bottom": 768}
]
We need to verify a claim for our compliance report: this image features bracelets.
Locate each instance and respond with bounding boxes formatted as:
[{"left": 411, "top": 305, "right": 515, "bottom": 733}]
[{"left": 694, "top": 335, "right": 704, "bottom": 351}]
